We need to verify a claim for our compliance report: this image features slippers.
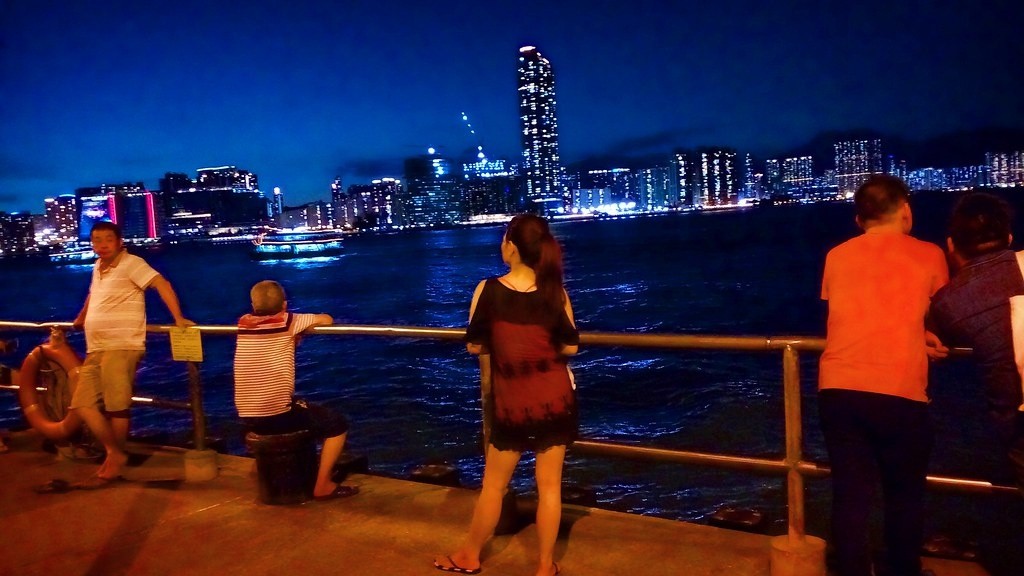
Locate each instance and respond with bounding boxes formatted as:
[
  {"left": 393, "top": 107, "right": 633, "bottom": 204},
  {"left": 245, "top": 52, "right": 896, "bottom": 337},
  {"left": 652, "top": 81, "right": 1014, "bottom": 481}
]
[
  {"left": 314, "top": 486, "right": 360, "bottom": 502},
  {"left": 434, "top": 555, "right": 482, "bottom": 575},
  {"left": 552, "top": 561, "right": 560, "bottom": 576}
]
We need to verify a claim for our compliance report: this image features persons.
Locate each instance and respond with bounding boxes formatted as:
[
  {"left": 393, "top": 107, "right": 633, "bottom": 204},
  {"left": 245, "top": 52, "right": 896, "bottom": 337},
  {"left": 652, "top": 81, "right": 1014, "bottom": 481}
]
[
  {"left": 922, "top": 188, "right": 1023, "bottom": 576},
  {"left": 232, "top": 279, "right": 360, "bottom": 502},
  {"left": 69, "top": 220, "right": 196, "bottom": 481},
  {"left": 811, "top": 172, "right": 951, "bottom": 576},
  {"left": 430, "top": 213, "right": 581, "bottom": 576}
]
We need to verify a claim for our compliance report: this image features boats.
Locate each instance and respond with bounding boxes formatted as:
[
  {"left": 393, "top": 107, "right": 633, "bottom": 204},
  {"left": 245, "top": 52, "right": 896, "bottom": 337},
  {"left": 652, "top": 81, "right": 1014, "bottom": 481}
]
[
  {"left": 49, "top": 248, "right": 97, "bottom": 266},
  {"left": 248, "top": 239, "right": 345, "bottom": 260}
]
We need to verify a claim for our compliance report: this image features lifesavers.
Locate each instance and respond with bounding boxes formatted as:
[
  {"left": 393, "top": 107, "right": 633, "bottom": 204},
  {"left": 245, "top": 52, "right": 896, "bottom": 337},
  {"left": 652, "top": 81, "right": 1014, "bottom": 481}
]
[{"left": 19, "top": 342, "right": 83, "bottom": 439}]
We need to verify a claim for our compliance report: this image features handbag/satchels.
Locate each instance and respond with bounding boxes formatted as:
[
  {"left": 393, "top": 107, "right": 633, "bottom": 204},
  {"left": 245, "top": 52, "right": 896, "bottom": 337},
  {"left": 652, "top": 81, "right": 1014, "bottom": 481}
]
[{"left": 1010, "top": 250, "right": 1024, "bottom": 413}]
